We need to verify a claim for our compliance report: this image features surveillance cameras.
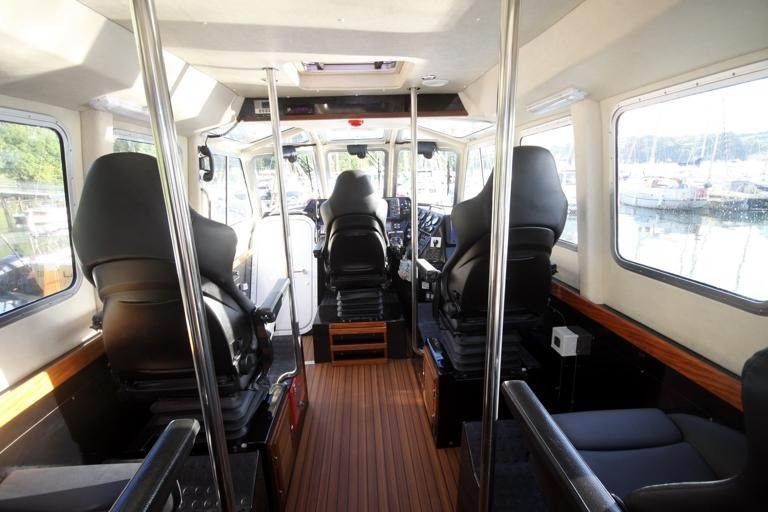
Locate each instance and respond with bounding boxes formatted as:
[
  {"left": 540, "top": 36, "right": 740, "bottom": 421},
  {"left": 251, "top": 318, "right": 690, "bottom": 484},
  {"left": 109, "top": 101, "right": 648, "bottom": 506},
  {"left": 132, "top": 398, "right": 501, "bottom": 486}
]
[
  {"left": 424, "top": 151, "right": 433, "bottom": 159},
  {"left": 288, "top": 155, "right": 299, "bottom": 163},
  {"left": 356, "top": 152, "right": 366, "bottom": 158}
]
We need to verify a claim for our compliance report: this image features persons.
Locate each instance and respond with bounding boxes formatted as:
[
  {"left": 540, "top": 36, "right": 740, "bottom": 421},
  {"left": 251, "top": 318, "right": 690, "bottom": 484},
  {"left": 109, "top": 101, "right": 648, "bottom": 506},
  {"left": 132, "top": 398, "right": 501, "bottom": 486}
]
[{"left": 690, "top": 157, "right": 713, "bottom": 200}]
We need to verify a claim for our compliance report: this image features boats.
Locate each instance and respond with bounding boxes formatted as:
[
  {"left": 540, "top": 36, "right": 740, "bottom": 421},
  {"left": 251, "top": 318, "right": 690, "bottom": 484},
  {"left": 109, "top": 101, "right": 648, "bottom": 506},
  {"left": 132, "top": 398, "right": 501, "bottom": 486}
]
[
  {"left": 14, "top": 194, "right": 70, "bottom": 233},
  {"left": 257, "top": 145, "right": 456, "bottom": 216},
  {"left": 0, "top": 247, "right": 75, "bottom": 316}
]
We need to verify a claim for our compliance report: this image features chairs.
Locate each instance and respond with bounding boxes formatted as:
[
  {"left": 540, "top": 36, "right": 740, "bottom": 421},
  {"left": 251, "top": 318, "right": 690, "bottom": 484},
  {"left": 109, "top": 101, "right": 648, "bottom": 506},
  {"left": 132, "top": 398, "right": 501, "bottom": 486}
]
[
  {"left": 73, "top": 152, "right": 290, "bottom": 399},
  {"left": 1, "top": 416, "right": 201, "bottom": 512},
  {"left": 416, "top": 145, "right": 568, "bottom": 340},
  {"left": 499, "top": 347, "right": 768, "bottom": 512},
  {"left": 320, "top": 169, "right": 390, "bottom": 266}
]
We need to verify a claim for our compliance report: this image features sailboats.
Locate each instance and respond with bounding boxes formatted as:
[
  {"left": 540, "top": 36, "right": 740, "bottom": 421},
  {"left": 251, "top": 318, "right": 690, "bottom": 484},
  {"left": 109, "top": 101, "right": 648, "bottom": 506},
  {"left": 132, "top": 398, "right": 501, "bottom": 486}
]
[{"left": 551, "top": 126, "right": 768, "bottom": 222}]
